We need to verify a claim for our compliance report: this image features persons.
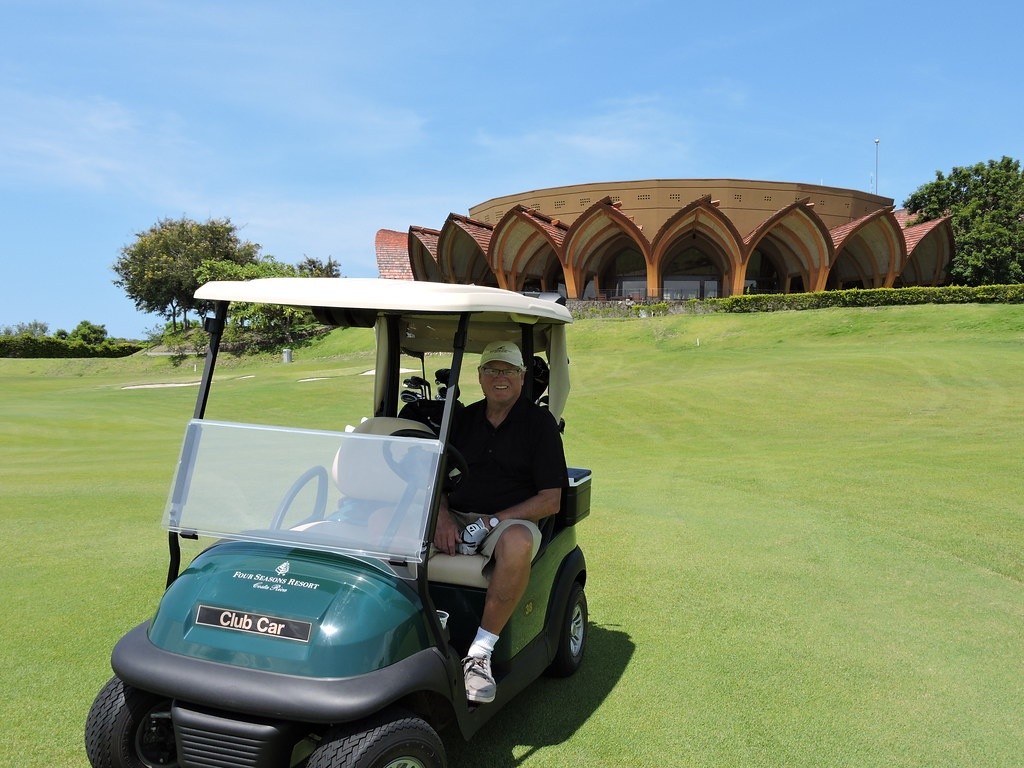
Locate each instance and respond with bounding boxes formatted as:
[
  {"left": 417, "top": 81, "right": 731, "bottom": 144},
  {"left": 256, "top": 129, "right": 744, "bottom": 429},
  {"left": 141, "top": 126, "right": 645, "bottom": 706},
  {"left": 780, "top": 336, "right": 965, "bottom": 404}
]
[{"left": 365, "top": 338, "right": 572, "bottom": 705}]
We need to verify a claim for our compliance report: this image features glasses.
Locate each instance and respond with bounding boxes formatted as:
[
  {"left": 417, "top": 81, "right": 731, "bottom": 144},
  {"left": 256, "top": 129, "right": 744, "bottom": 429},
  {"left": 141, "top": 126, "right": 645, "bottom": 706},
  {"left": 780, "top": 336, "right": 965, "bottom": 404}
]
[{"left": 482, "top": 367, "right": 521, "bottom": 376}]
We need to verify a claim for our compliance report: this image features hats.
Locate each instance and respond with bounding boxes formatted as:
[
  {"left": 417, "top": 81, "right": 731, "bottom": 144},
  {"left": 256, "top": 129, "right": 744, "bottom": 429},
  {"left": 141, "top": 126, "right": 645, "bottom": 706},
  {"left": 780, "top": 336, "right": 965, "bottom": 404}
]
[
  {"left": 480, "top": 340, "right": 524, "bottom": 370},
  {"left": 456, "top": 517, "right": 489, "bottom": 554}
]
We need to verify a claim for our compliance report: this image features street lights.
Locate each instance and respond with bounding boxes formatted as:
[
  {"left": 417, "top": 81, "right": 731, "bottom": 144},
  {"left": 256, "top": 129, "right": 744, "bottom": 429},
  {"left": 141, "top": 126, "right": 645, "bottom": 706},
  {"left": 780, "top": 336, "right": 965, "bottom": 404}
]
[{"left": 874, "top": 139, "right": 879, "bottom": 195}]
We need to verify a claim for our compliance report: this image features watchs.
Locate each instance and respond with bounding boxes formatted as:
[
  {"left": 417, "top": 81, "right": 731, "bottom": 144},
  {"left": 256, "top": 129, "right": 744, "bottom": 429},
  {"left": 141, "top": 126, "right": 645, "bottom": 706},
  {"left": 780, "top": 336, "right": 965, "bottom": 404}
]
[{"left": 486, "top": 514, "right": 499, "bottom": 529}]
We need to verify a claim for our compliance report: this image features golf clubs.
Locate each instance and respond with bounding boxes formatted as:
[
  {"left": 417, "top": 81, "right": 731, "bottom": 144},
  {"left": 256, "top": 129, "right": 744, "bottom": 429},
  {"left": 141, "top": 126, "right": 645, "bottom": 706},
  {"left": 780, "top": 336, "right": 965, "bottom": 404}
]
[{"left": 400, "top": 375, "right": 431, "bottom": 404}]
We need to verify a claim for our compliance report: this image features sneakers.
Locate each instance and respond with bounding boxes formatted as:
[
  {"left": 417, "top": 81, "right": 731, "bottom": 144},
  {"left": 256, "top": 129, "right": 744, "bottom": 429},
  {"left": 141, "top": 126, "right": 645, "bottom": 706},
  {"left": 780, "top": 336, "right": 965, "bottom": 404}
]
[{"left": 463, "top": 657, "right": 496, "bottom": 702}]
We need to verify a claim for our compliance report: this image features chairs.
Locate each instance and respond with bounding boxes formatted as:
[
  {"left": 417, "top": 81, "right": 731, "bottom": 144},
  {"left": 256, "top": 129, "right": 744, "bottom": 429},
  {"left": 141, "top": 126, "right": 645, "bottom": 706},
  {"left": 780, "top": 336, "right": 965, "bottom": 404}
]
[
  {"left": 403, "top": 537, "right": 492, "bottom": 592},
  {"left": 291, "top": 415, "right": 435, "bottom": 581}
]
